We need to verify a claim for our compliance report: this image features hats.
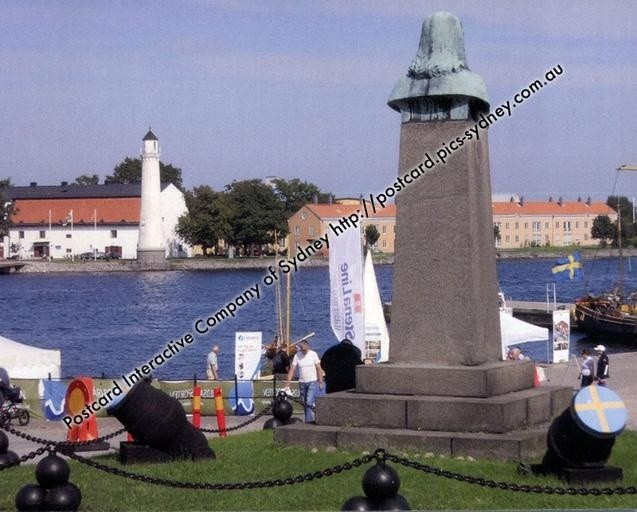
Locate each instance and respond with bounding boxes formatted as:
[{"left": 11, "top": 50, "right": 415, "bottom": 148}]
[{"left": 592, "top": 344, "right": 605, "bottom": 352}]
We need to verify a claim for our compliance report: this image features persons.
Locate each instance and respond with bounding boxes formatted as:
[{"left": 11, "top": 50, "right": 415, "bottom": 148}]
[
  {"left": 577, "top": 348, "right": 594, "bottom": 387},
  {"left": 320, "top": 339, "right": 365, "bottom": 394},
  {"left": 507, "top": 348, "right": 520, "bottom": 360},
  {"left": 286, "top": 339, "right": 322, "bottom": 422},
  {"left": 205, "top": 345, "right": 220, "bottom": 381},
  {"left": 592, "top": 344, "right": 610, "bottom": 387}
]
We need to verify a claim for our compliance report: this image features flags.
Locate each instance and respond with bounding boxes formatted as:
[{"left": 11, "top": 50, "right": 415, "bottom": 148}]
[{"left": 550, "top": 252, "right": 585, "bottom": 283}]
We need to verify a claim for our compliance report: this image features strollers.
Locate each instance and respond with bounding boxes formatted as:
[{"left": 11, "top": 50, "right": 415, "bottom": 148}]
[{"left": 1, "top": 367, "right": 30, "bottom": 425}]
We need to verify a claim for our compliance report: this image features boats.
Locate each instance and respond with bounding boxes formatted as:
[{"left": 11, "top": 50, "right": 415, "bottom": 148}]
[{"left": 573, "top": 165, "right": 637, "bottom": 340}]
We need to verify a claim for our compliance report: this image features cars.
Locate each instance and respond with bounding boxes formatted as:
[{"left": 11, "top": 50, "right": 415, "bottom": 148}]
[{"left": 81, "top": 251, "right": 122, "bottom": 259}]
